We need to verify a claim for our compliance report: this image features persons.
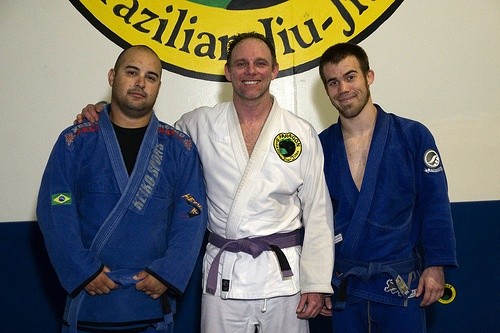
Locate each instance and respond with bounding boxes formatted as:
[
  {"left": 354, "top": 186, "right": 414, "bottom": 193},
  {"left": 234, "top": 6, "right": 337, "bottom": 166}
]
[
  {"left": 314, "top": 42, "right": 459, "bottom": 333},
  {"left": 35, "top": 44, "right": 207, "bottom": 333},
  {"left": 74, "top": 33, "right": 334, "bottom": 332}
]
